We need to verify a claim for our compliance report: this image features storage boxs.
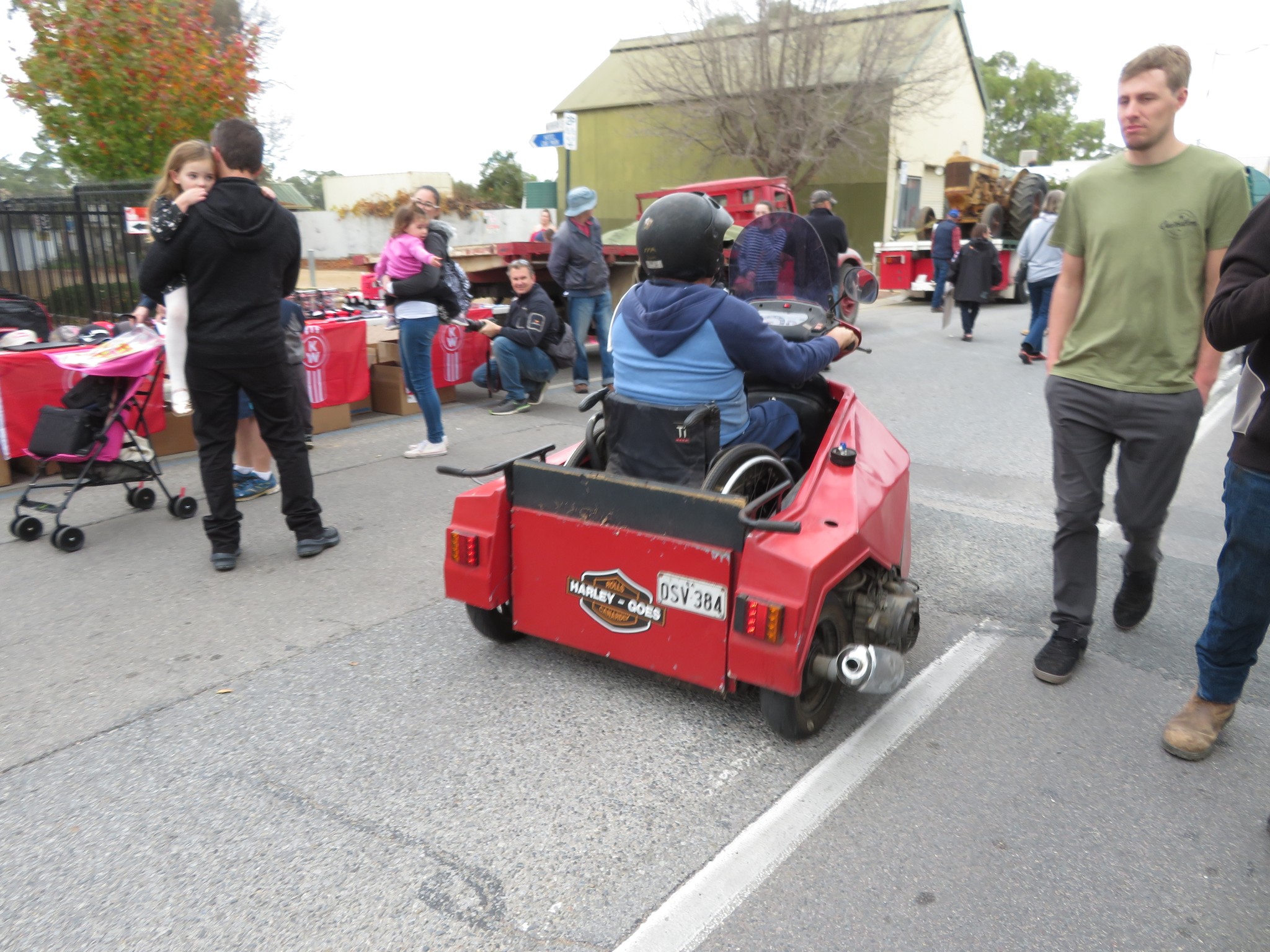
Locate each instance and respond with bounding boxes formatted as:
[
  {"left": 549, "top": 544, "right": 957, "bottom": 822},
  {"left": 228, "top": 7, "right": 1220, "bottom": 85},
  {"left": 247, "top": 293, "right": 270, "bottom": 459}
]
[{"left": 11, "top": 341, "right": 457, "bottom": 476}]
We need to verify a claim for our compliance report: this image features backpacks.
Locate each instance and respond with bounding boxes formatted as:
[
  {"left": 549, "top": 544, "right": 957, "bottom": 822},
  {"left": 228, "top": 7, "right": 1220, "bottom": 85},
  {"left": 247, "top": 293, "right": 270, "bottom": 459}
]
[
  {"left": 426, "top": 233, "right": 473, "bottom": 326},
  {"left": 28, "top": 405, "right": 92, "bottom": 454}
]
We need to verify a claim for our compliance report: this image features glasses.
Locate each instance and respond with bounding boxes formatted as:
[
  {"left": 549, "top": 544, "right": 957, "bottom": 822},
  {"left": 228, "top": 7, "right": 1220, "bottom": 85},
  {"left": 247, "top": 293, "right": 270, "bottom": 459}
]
[
  {"left": 411, "top": 199, "right": 441, "bottom": 211},
  {"left": 509, "top": 259, "right": 530, "bottom": 266}
]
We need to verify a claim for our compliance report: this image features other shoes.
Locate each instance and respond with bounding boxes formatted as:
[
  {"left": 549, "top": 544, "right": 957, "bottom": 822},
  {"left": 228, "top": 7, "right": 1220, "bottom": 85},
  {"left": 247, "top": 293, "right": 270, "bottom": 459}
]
[
  {"left": 450, "top": 315, "right": 470, "bottom": 326},
  {"left": 931, "top": 305, "right": 945, "bottom": 313},
  {"left": 1029, "top": 352, "right": 1048, "bottom": 361},
  {"left": 574, "top": 383, "right": 588, "bottom": 393},
  {"left": 961, "top": 334, "right": 974, "bottom": 342},
  {"left": 1018, "top": 351, "right": 1033, "bottom": 365},
  {"left": 170, "top": 389, "right": 194, "bottom": 418},
  {"left": 304, "top": 435, "right": 313, "bottom": 448},
  {"left": 604, "top": 383, "right": 614, "bottom": 391},
  {"left": 384, "top": 315, "right": 401, "bottom": 331}
]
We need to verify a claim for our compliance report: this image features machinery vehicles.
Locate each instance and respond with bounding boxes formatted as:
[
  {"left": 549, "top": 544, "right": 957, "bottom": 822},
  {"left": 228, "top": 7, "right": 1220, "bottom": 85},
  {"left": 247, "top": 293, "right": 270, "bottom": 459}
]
[{"left": 915, "top": 151, "right": 1050, "bottom": 248}]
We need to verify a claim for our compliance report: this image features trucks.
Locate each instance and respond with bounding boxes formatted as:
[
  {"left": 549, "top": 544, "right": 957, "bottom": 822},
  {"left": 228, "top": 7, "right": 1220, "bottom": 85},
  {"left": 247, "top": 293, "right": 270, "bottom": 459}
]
[
  {"left": 352, "top": 176, "right": 865, "bottom": 325},
  {"left": 873, "top": 236, "right": 1031, "bottom": 304}
]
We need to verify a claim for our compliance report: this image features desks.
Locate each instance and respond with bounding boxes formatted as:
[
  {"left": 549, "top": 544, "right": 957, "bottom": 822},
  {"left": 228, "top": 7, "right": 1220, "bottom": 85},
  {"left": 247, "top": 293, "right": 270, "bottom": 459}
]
[{"left": 0, "top": 304, "right": 511, "bottom": 486}]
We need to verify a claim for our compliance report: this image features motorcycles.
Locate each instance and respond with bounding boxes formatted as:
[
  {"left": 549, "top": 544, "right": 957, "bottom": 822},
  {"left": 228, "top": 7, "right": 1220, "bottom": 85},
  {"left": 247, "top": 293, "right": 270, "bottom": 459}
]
[{"left": 433, "top": 208, "right": 922, "bottom": 743}]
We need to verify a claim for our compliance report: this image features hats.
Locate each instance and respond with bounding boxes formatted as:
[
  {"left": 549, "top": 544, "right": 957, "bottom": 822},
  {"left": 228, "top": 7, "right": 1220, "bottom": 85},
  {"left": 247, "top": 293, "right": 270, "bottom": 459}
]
[
  {"left": 47, "top": 317, "right": 167, "bottom": 346},
  {"left": 0, "top": 329, "right": 40, "bottom": 348},
  {"left": 808, "top": 189, "right": 839, "bottom": 204},
  {"left": 947, "top": 209, "right": 959, "bottom": 218},
  {"left": 564, "top": 186, "right": 598, "bottom": 216}
]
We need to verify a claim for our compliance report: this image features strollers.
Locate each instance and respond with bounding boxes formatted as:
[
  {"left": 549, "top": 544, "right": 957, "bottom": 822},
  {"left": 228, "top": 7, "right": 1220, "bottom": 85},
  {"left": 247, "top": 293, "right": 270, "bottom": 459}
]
[{"left": 8, "top": 309, "right": 198, "bottom": 554}]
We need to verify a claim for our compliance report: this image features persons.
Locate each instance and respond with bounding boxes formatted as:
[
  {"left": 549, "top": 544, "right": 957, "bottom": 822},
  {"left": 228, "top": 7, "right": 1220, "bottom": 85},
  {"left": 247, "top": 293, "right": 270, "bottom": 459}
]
[
  {"left": 604, "top": 191, "right": 859, "bottom": 517},
  {"left": 785, "top": 191, "right": 849, "bottom": 372},
  {"left": 929, "top": 188, "right": 1070, "bottom": 363},
  {"left": 738, "top": 202, "right": 787, "bottom": 301},
  {"left": 1033, "top": 43, "right": 1252, "bottom": 685},
  {"left": 1164, "top": 196, "right": 1270, "bottom": 762},
  {"left": 131, "top": 121, "right": 610, "bottom": 570}
]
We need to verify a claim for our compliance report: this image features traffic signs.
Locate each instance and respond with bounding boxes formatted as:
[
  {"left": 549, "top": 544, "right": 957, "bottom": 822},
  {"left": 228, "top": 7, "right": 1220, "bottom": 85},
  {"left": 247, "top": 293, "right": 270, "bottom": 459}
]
[{"left": 529, "top": 130, "right": 564, "bottom": 148}]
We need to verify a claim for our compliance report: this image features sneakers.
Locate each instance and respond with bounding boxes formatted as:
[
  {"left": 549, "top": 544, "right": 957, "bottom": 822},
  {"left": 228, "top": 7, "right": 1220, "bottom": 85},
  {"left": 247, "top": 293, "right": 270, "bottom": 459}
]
[
  {"left": 1032, "top": 629, "right": 1088, "bottom": 684},
  {"left": 231, "top": 463, "right": 281, "bottom": 501},
  {"left": 1162, "top": 687, "right": 1239, "bottom": 760},
  {"left": 402, "top": 435, "right": 449, "bottom": 458},
  {"left": 296, "top": 526, "right": 340, "bottom": 557},
  {"left": 1113, "top": 567, "right": 1156, "bottom": 629},
  {"left": 211, "top": 545, "right": 242, "bottom": 569},
  {"left": 527, "top": 382, "right": 548, "bottom": 405},
  {"left": 489, "top": 398, "right": 530, "bottom": 415}
]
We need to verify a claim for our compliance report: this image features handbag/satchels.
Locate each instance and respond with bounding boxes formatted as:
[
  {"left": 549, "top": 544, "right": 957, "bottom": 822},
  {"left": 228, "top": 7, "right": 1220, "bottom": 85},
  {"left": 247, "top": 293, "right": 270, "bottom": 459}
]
[
  {"left": 0, "top": 286, "right": 53, "bottom": 346},
  {"left": 541, "top": 315, "right": 577, "bottom": 369},
  {"left": 60, "top": 374, "right": 126, "bottom": 446},
  {"left": 1013, "top": 262, "right": 1028, "bottom": 285},
  {"left": 76, "top": 429, "right": 154, "bottom": 480}
]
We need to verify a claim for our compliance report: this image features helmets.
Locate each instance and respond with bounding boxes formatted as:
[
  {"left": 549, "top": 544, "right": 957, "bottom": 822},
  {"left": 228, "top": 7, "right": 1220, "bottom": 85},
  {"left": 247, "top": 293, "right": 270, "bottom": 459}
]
[{"left": 636, "top": 191, "right": 735, "bottom": 282}]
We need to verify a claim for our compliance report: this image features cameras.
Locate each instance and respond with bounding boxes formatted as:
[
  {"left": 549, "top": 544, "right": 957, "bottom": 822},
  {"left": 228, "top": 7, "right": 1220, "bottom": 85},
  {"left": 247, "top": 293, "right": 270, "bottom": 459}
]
[{"left": 465, "top": 316, "right": 507, "bottom": 339}]
